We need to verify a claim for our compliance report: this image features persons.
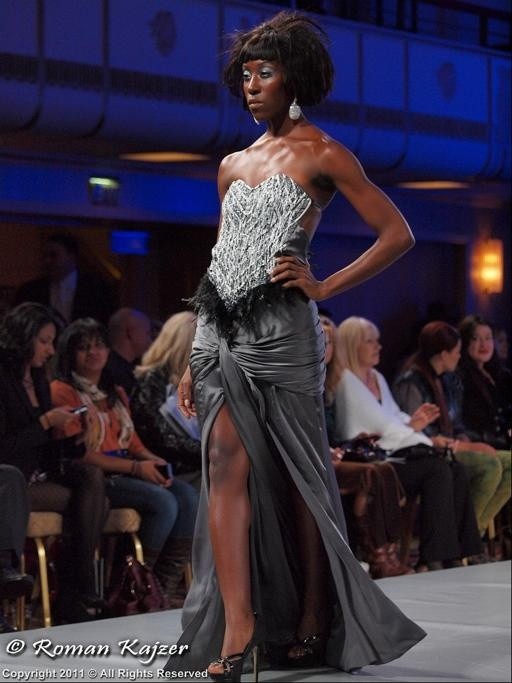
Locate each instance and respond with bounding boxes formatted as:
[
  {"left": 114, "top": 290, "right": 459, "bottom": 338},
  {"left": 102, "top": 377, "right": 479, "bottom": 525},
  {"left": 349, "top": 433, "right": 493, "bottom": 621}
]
[
  {"left": 1, "top": 231, "right": 512, "bottom": 633},
  {"left": 161, "top": 14, "right": 427, "bottom": 683}
]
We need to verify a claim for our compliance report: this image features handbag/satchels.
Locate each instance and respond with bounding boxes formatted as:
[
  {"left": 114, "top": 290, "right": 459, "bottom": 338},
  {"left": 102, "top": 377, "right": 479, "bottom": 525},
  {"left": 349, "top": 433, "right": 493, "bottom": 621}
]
[{"left": 341, "top": 434, "right": 386, "bottom": 461}]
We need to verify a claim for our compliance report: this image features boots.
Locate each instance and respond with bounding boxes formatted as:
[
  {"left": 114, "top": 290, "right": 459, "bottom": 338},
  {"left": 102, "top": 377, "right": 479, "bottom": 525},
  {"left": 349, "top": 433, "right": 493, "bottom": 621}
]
[
  {"left": 368, "top": 546, "right": 403, "bottom": 578},
  {"left": 384, "top": 542, "right": 416, "bottom": 573}
]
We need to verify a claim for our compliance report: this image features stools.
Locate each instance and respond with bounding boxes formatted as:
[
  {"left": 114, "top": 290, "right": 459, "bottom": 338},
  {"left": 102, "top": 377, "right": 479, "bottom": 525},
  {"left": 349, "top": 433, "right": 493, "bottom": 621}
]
[{"left": 0, "top": 437, "right": 511, "bottom": 629}]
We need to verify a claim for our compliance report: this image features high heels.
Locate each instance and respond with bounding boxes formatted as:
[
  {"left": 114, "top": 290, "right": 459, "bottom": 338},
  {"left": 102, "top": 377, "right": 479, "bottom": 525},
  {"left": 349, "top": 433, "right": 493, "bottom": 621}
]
[
  {"left": 208, "top": 613, "right": 268, "bottom": 681},
  {"left": 285, "top": 606, "right": 332, "bottom": 664}
]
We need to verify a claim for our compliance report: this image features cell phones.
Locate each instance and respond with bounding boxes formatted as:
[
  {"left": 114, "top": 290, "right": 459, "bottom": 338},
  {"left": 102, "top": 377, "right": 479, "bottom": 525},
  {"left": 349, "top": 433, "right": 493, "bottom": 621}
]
[
  {"left": 155, "top": 463, "right": 174, "bottom": 480},
  {"left": 70, "top": 404, "right": 89, "bottom": 415}
]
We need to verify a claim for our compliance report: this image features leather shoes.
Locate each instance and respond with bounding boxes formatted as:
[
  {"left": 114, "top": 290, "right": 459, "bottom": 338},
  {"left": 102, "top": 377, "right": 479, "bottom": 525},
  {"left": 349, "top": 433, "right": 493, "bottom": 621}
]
[{"left": 79, "top": 591, "right": 104, "bottom": 607}]
